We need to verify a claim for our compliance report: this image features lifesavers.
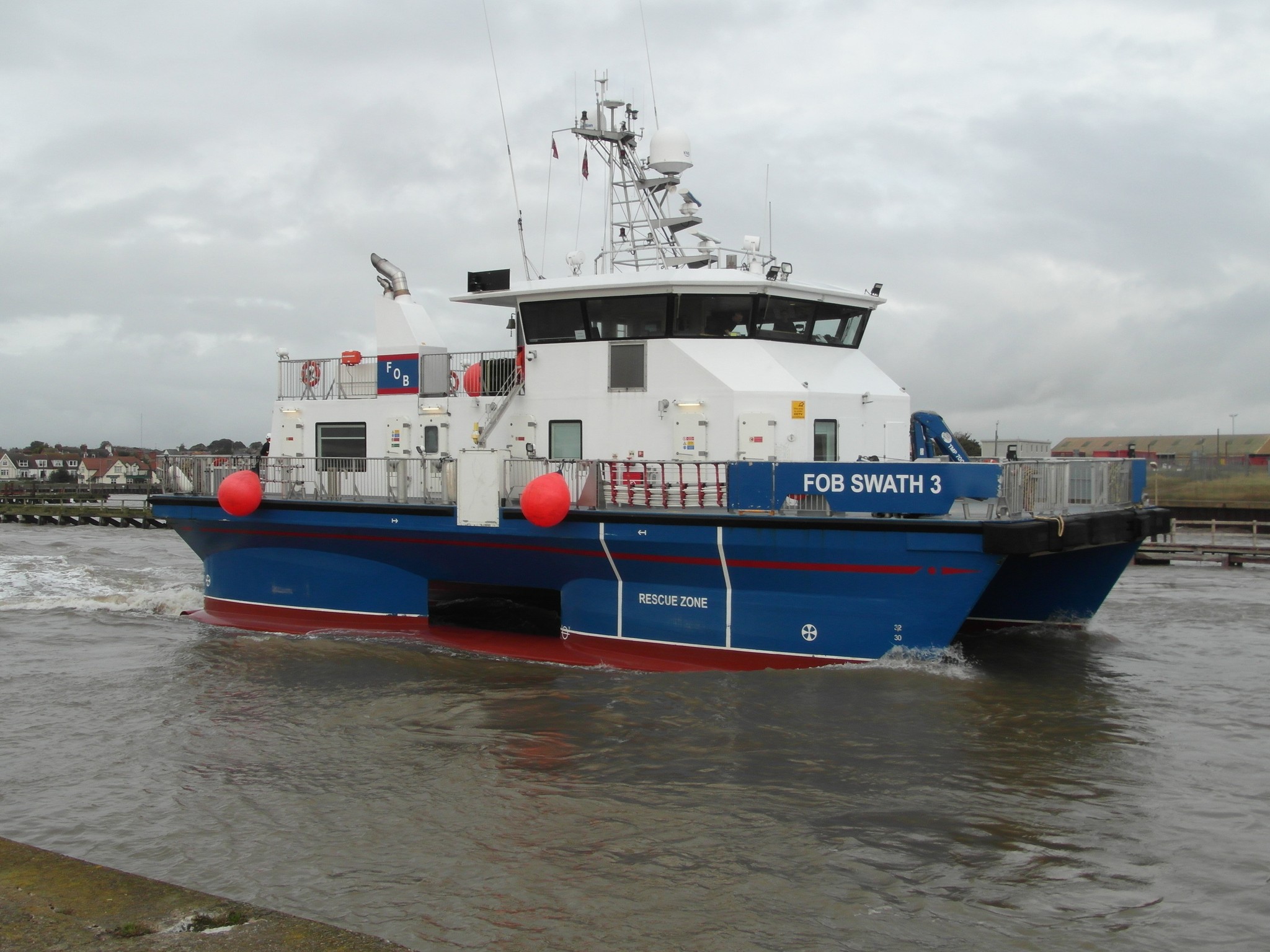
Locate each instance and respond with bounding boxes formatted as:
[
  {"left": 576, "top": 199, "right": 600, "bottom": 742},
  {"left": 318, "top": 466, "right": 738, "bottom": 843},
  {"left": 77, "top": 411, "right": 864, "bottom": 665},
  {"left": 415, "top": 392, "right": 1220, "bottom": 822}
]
[
  {"left": 983, "top": 459, "right": 998, "bottom": 462},
  {"left": 301, "top": 360, "right": 320, "bottom": 387},
  {"left": 788, "top": 494, "right": 806, "bottom": 500},
  {"left": 450, "top": 372, "right": 459, "bottom": 394},
  {"left": 517, "top": 350, "right": 525, "bottom": 378}
]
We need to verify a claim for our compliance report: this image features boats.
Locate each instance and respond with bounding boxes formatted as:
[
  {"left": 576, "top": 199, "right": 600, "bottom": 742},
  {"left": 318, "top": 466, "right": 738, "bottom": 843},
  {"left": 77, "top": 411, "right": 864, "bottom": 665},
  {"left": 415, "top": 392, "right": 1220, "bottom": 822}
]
[{"left": 145, "top": 1, "right": 1179, "bottom": 669}]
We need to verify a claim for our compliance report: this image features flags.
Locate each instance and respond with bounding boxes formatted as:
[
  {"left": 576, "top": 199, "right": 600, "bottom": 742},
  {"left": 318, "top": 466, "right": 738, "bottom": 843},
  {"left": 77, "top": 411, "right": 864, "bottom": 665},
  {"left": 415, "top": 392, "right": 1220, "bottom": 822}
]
[
  {"left": 551, "top": 140, "right": 559, "bottom": 159},
  {"left": 582, "top": 150, "right": 589, "bottom": 181}
]
